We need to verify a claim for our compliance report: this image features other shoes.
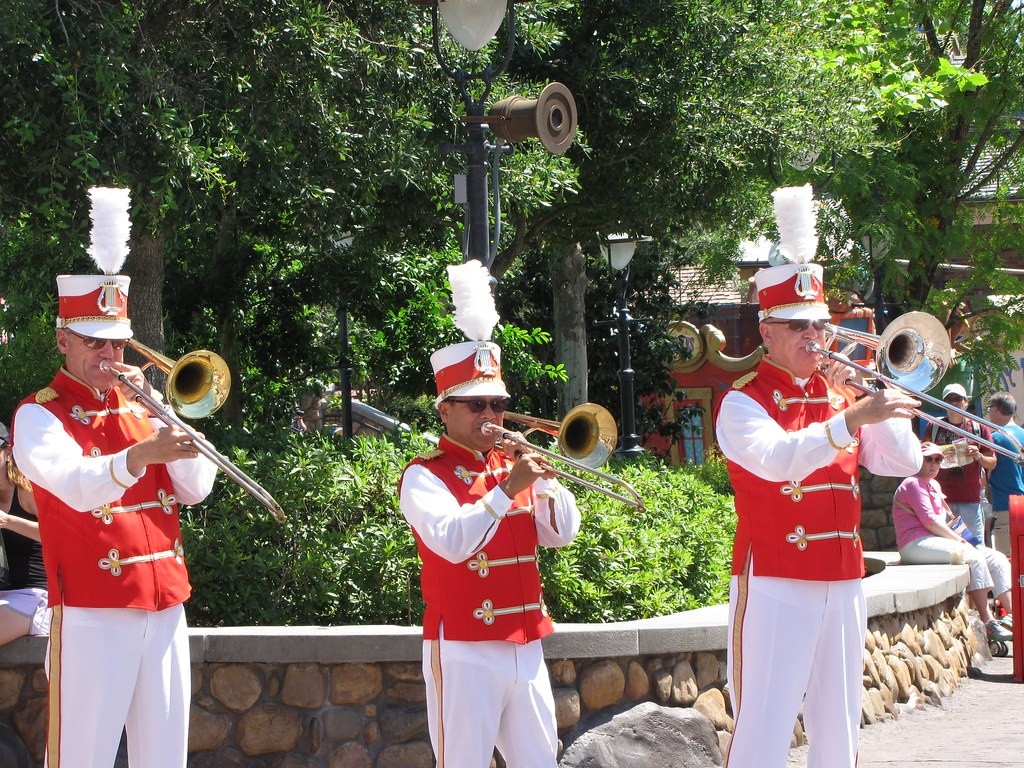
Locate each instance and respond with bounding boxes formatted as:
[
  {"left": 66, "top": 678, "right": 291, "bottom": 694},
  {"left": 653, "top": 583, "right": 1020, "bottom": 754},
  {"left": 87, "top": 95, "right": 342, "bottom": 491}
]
[
  {"left": 1001, "top": 614, "right": 1012, "bottom": 632},
  {"left": 985, "top": 619, "right": 1012, "bottom": 641}
]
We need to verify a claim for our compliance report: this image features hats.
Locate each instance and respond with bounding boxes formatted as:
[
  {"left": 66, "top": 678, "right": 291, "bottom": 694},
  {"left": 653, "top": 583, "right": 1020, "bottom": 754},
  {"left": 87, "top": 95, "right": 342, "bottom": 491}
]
[
  {"left": 431, "top": 342, "right": 511, "bottom": 409},
  {"left": 943, "top": 384, "right": 966, "bottom": 400},
  {"left": 755, "top": 263, "right": 832, "bottom": 321},
  {"left": 921, "top": 442, "right": 944, "bottom": 458},
  {"left": 57, "top": 275, "right": 133, "bottom": 338}
]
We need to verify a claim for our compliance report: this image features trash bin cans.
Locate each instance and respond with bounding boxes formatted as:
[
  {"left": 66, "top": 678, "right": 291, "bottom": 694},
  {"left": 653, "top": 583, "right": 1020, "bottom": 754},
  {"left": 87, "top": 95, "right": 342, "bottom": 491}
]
[{"left": 1008, "top": 494, "right": 1024, "bottom": 684}]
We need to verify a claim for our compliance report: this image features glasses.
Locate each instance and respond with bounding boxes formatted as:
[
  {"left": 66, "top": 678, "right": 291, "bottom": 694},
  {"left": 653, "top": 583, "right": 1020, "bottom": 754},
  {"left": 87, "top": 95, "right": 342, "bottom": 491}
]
[
  {"left": 946, "top": 397, "right": 962, "bottom": 402},
  {"left": 69, "top": 331, "right": 130, "bottom": 350},
  {"left": 445, "top": 399, "right": 509, "bottom": 412},
  {"left": 769, "top": 319, "right": 827, "bottom": 330},
  {"left": 987, "top": 406, "right": 995, "bottom": 412},
  {"left": 926, "top": 456, "right": 940, "bottom": 464}
]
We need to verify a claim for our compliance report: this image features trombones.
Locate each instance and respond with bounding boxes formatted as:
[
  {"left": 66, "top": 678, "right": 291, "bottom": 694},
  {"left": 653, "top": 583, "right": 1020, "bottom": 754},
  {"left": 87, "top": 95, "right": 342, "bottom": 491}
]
[
  {"left": 99, "top": 338, "right": 287, "bottom": 523},
  {"left": 805, "top": 310, "right": 1023, "bottom": 467},
  {"left": 481, "top": 402, "right": 646, "bottom": 512}
]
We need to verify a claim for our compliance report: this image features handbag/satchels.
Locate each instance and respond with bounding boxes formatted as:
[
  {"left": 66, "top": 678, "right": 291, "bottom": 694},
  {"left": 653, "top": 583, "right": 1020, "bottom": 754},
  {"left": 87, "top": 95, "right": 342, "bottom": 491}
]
[{"left": 947, "top": 515, "right": 982, "bottom": 546}]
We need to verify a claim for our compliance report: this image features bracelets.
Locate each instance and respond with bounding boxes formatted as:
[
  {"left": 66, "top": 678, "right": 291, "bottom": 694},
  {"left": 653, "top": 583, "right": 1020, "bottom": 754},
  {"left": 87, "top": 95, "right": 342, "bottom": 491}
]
[
  {"left": 978, "top": 453, "right": 983, "bottom": 463},
  {"left": 961, "top": 539, "right": 966, "bottom": 544}
]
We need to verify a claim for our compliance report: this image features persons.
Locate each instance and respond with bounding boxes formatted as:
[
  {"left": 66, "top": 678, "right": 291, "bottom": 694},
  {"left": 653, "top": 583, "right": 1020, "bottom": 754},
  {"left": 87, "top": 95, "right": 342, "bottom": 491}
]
[
  {"left": 0, "top": 423, "right": 53, "bottom": 645},
  {"left": 11, "top": 275, "right": 218, "bottom": 768},
  {"left": 921, "top": 383, "right": 1024, "bottom": 611},
  {"left": 893, "top": 441, "right": 1013, "bottom": 641},
  {"left": 399, "top": 341, "right": 583, "bottom": 768},
  {"left": 292, "top": 399, "right": 307, "bottom": 432},
  {"left": 714, "top": 264, "right": 924, "bottom": 768}
]
